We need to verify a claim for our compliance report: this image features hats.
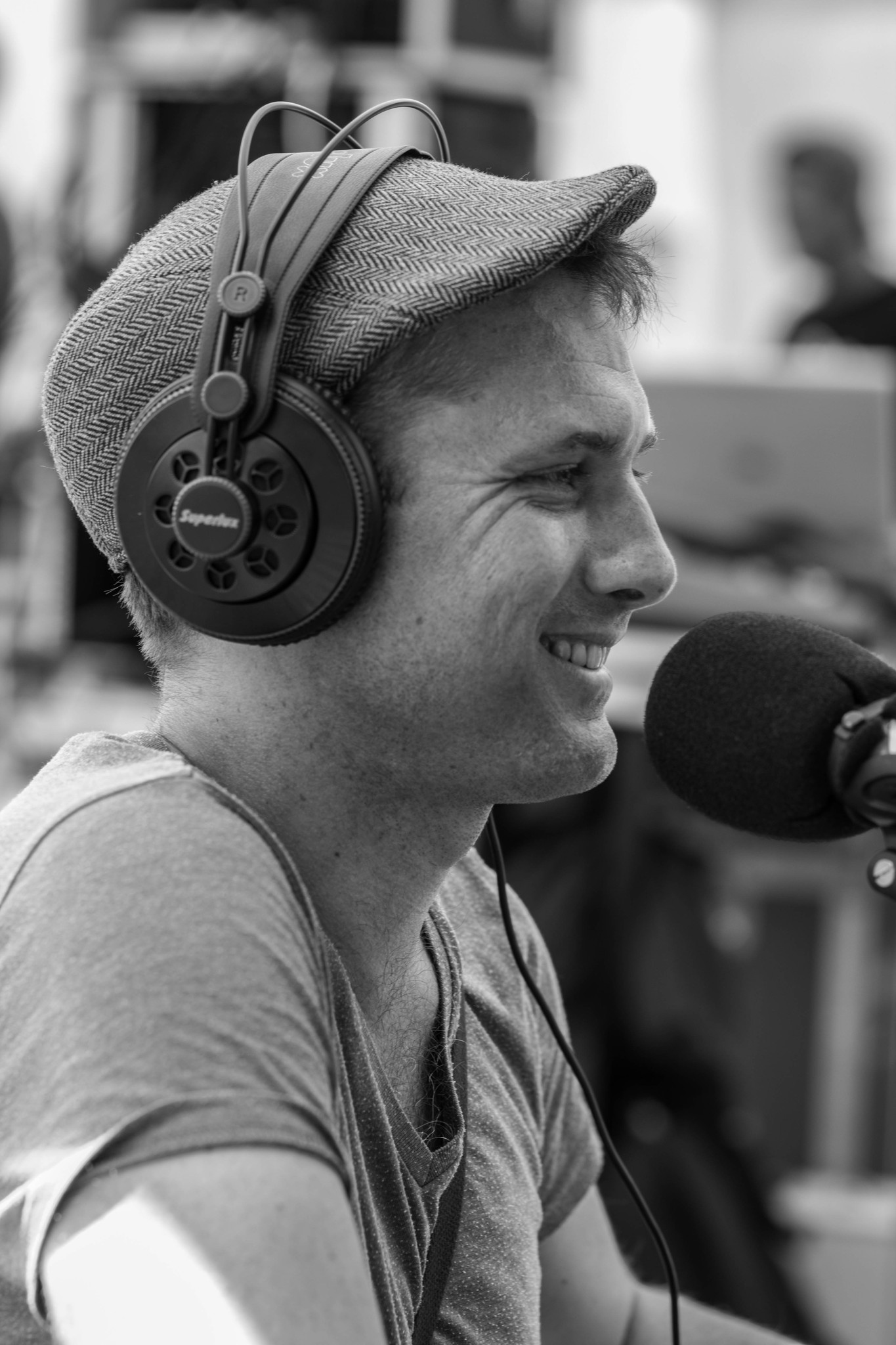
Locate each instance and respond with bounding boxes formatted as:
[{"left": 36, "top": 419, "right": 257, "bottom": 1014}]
[{"left": 43, "top": 159, "right": 658, "bottom": 573}]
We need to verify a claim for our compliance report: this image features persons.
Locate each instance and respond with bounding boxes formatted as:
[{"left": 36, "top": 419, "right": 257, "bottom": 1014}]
[
  {"left": 776, "top": 146, "right": 894, "bottom": 350},
  {"left": 0, "top": 101, "right": 834, "bottom": 1345}
]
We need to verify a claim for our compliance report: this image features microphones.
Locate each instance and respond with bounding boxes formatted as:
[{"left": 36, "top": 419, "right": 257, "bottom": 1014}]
[{"left": 643, "top": 612, "right": 896, "bottom": 844}]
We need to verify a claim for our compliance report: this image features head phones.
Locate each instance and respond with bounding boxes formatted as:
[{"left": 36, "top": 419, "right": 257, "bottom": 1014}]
[{"left": 120, "top": 99, "right": 454, "bottom": 648}]
